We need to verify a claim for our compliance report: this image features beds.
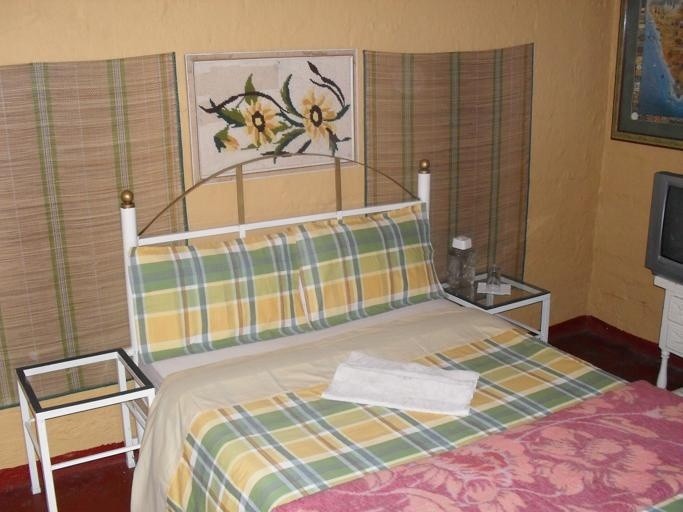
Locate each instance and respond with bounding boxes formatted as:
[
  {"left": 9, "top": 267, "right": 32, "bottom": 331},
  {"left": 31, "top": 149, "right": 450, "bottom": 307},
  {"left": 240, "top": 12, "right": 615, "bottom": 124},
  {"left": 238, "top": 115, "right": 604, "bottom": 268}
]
[{"left": 116, "top": 156, "right": 683, "bottom": 512}]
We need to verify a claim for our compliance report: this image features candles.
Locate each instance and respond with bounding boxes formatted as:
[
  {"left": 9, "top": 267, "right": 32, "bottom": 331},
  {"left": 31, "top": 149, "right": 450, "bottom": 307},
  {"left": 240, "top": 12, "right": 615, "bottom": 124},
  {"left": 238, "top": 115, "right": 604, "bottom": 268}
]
[{"left": 452, "top": 235, "right": 472, "bottom": 251}]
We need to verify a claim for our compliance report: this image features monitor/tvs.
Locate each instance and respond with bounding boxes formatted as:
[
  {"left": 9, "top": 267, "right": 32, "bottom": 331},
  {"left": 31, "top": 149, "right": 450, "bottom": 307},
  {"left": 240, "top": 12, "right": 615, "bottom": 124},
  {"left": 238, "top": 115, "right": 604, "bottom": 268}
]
[{"left": 644, "top": 171, "right": 683, "bottom": 284}]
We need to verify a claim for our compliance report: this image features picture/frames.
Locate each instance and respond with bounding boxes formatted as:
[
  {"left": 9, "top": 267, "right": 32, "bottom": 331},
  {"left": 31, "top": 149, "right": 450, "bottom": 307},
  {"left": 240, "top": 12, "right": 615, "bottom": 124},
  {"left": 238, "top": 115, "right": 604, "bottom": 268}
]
[
  {"left": 608, "top": 1, "right": 682, "bottom": 151},
  {"left": 182, "top": 49, "right": 359, "bottom": 186}
]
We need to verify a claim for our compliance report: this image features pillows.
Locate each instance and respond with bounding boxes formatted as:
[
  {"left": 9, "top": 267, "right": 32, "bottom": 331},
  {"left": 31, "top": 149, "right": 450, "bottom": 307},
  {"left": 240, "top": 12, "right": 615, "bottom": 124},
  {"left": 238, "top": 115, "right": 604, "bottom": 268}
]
[
  {"left": 289, "top": 202, "right": 446, "bottom": 331},
  {"left": 128, "top": 229, "right": 313, "bottom": 359}
]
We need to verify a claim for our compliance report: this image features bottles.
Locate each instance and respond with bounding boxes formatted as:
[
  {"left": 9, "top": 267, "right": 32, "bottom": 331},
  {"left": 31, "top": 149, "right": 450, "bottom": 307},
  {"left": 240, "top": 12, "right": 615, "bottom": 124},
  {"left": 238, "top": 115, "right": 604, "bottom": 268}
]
[
  {"left": 447, "top": 234, "right": 476, "bottom": 292},
  {"left": 486, "top": 263, "right": 501, "bottom": 294}
]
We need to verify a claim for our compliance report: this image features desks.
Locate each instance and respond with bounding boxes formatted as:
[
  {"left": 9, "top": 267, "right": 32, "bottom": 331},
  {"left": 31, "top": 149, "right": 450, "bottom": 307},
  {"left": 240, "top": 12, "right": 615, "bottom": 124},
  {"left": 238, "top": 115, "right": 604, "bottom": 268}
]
[
  {"left": 652, "top": 273, "right": 683, "bottom": 390},
  {"left": 439, "top": 270, "right": 552, "bottom": 344},
  {"left": 16, "top": 347, "right": 157, "bottom": 512}
]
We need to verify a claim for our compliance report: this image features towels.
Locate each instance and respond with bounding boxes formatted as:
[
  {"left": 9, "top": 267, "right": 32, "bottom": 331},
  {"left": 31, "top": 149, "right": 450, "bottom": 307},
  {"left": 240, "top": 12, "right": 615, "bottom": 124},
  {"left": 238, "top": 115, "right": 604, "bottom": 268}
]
[{"left": 323, "top": 350, "right": 480, "bottom": 418}]
